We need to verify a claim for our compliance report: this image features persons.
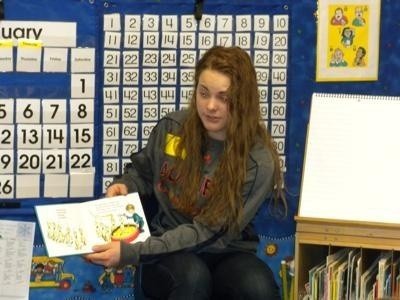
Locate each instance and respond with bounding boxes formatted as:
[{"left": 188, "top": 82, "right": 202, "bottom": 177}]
[{"left": 84, "top": 46, "right": 288, "bottom": 300}]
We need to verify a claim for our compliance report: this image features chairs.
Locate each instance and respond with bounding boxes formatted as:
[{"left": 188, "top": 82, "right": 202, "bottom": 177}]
[{"left": 135, "top": 194, "right": 160, "bottom": 300}]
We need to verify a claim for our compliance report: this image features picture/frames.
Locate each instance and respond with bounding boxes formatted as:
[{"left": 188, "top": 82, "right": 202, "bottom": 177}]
[{"left": 315, "top": 0, "right": 382, "bottom": 83}]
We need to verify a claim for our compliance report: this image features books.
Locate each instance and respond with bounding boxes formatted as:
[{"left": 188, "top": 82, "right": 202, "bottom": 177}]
[{"left": 297, "top": 247, "right": 400, "bottom": 300}]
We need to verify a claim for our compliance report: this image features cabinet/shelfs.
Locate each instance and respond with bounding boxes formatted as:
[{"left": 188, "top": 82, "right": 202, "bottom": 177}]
[{"left": 293, "top": 215, "right": 400, "bottom": 300}]
